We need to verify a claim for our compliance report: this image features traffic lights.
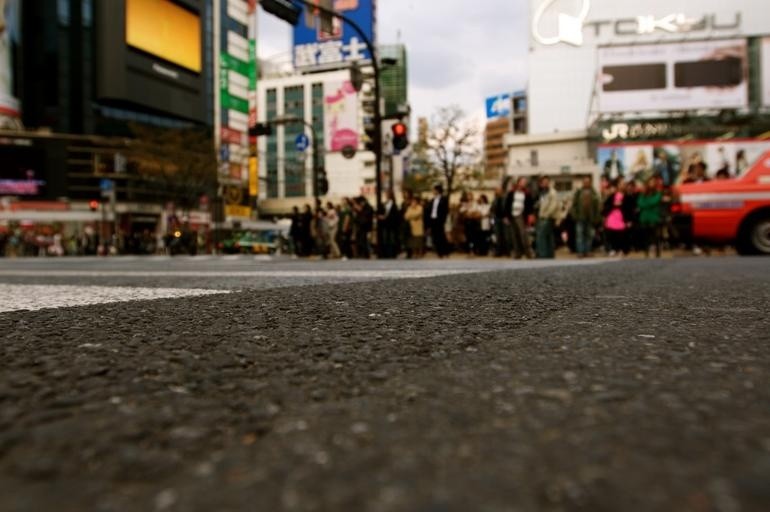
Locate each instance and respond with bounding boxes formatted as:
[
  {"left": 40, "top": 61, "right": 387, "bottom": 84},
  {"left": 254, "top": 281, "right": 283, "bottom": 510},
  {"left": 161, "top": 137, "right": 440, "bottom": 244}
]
[
  {"left": 363, "top": 127, "right": 376, "bottom": 154},
  {"left": 89, "top": 200, "right": 98, "bottom": 211},
  {"left": 391, "top": 123, "right": 408, "bottom": 150}
]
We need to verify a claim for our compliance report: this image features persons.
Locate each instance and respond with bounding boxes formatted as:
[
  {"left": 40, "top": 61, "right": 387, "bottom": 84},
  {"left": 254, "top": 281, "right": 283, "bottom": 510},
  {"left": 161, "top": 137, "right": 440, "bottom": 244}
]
[
  {"left": 602, "top": 148, "right": 625, "bottom": 183},
  {"left": 0, "top": 202, "right": 276, "bottom": 258},
  {"left": 276, "top": 171, "right": 671, "bottom": 261},
  {"left": 627, "top": 148, "right": 651, "bottom": 181},
  {"left": 651, "top": 145, "right": 751, "bottom": 187}
]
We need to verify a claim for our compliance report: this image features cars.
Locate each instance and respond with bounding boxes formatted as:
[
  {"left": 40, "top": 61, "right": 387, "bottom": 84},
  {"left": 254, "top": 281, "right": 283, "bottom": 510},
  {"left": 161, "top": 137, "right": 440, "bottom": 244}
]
[
  {"left": 670, "top": 147, "right": 769, "bottom": 256},
  {"left": 218, "top": 229, "right": 288, "bottom": 254}
]
